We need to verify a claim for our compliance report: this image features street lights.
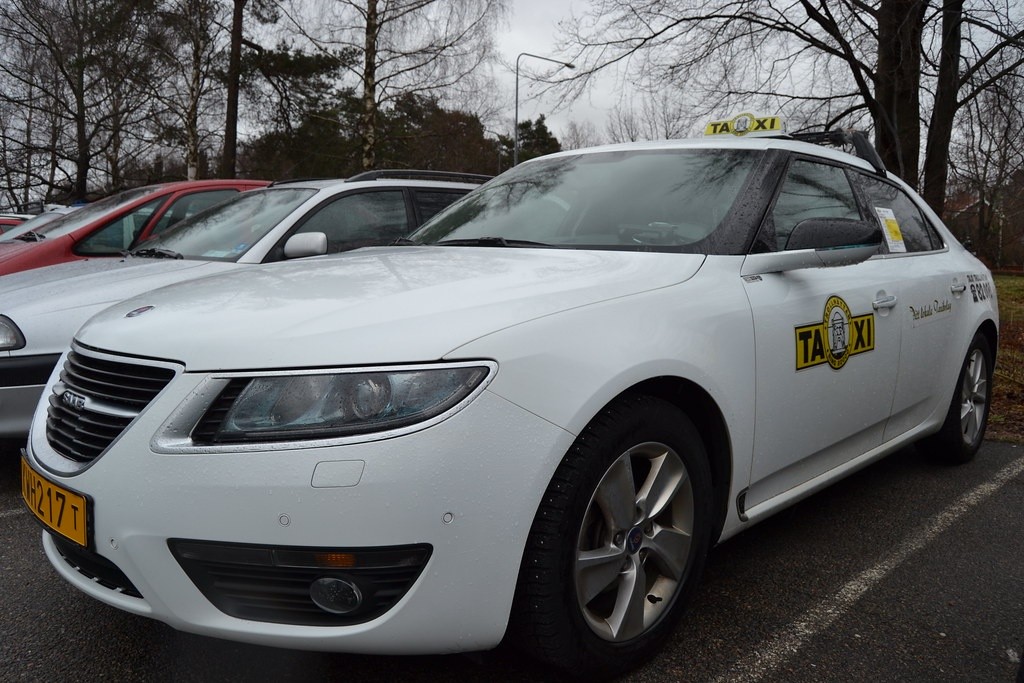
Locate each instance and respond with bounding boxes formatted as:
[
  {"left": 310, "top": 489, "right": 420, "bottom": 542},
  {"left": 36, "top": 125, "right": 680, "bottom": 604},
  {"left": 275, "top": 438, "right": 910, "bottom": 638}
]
[{"left": 513, "top": 51, "right": 581, "bottom": 178}]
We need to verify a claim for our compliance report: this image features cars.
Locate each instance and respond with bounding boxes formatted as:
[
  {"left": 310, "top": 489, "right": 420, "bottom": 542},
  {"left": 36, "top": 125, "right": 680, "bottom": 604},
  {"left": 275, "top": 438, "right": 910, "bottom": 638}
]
[
  {"left": 0, "top": 177, "right": 272, "bottom": 286},
  {"left": 0, "top": 164, "right": 499, "bottom": 445},
  {"left": 18, "top": 112, "right": 1002, "bottom": 668},
  {"left": 0, "top": 198, "right": 91, "bottom": 252}
]
